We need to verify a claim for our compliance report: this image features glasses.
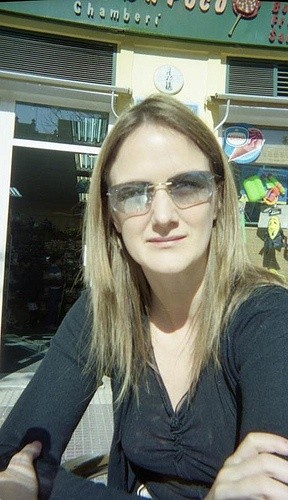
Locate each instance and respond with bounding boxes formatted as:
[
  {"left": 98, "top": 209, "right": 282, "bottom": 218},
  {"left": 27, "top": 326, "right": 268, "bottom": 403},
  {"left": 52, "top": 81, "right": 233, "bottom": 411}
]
[{"left": 109, "top": 171, "right": 217, "bottom": 216}]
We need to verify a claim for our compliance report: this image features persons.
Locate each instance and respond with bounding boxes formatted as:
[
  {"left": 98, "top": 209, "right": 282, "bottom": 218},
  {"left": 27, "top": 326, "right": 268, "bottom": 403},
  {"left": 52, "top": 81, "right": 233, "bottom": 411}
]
[{"left": 0, "top": 93, "right": 288, "bottom": 499}]
